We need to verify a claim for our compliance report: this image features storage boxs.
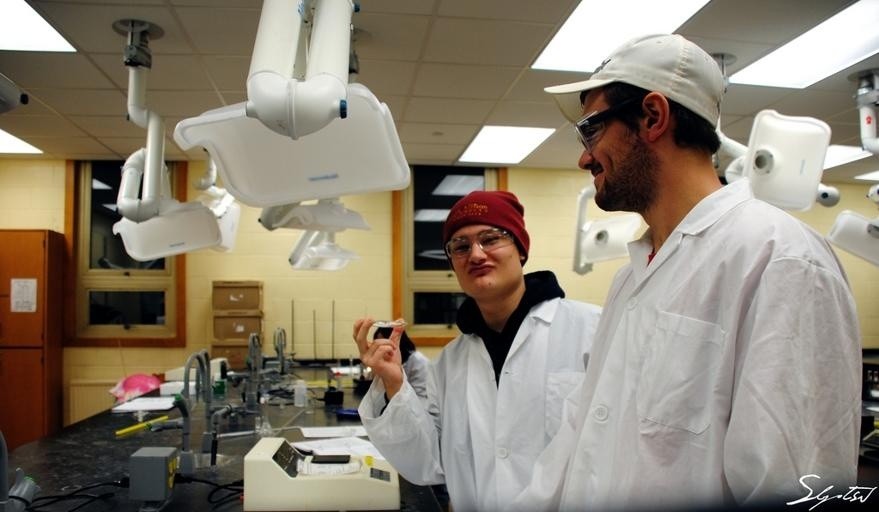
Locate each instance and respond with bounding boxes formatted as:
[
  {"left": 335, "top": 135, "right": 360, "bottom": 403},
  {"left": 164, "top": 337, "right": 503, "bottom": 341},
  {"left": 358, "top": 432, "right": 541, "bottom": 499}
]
[{"left": 205, "top": 279, "right": 265, "bottom": 369}]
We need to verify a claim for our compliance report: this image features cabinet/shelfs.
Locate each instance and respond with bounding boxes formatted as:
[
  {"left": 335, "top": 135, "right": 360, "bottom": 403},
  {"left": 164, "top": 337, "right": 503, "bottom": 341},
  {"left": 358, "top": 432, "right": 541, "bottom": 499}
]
[{"left": 0, "top": 229, "right": 64, "bottom": 450}]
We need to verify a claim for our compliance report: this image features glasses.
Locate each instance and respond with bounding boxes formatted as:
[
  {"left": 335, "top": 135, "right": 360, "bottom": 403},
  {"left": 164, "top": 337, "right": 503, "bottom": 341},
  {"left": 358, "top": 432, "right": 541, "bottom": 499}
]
[
  {"left": 446, "top": 228, "right": 513, "bottom": 257},
  {"left": 575, "top": 108, "right": 611, "bottom": 154}
]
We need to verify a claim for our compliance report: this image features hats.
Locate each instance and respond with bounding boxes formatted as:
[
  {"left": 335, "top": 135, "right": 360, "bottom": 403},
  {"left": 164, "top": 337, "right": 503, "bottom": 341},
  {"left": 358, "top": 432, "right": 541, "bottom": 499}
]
[
  {"left": 544, "top": 34, "right": 723, "bottom": 129},
  {"left": 444, "top": 191, "right": 529, "bottom": 271}
]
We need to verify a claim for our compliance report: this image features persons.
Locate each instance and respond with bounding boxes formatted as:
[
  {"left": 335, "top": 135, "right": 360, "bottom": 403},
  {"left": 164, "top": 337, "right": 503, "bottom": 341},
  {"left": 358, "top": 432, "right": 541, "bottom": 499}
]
[
  {"left": 507, "top": 37, "right": 861, "bottom": 512},
  {"left": 374, "top": 326, "right": 432, "bottom": 410},
  {"left": 352, "top": 192, "right": 604, "bottom": 512}
]
